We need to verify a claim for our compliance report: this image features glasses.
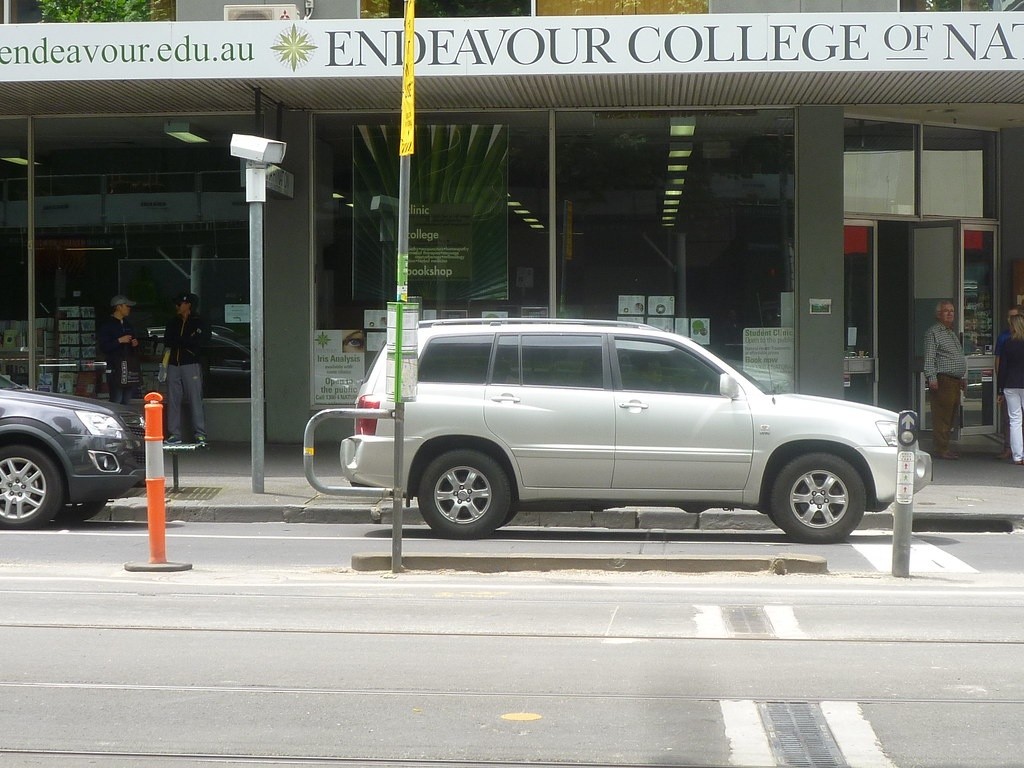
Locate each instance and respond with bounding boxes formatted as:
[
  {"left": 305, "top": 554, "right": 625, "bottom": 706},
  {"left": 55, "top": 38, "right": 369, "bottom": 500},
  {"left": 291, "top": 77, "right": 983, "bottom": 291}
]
[{"left": 178, "top": 302, "right": 184, "bottom": 307}]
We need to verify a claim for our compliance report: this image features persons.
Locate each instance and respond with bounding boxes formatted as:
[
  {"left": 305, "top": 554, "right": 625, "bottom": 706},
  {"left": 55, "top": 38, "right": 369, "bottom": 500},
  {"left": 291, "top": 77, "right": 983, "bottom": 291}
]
[
  {"left": 923, "top": 299, "right": 967, "bottom": 460},
  {"left": 99, "top": 293, "right": 141, "bottom": 405},
  {"left": 158, "top": 293, "right": 210, "bottom": 448},
  {"left": 995, "top": 305, "right": 1024, "bottom": 464}
]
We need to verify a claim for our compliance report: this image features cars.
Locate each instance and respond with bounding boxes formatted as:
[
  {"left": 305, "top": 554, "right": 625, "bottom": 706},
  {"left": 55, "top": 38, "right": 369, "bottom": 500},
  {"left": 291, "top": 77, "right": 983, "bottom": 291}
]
[
  {"left": 0, "top": 376, "right": 151, "bottom": 531},
  {"left": 148, "top": 324, "right": 251, "bottom": 399}
]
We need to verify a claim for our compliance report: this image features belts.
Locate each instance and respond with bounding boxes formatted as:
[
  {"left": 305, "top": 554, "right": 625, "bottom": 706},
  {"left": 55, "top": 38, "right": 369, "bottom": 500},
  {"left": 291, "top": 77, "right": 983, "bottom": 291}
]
[{"left": 941, "top": 374, "right": 961, "bottom": 380}]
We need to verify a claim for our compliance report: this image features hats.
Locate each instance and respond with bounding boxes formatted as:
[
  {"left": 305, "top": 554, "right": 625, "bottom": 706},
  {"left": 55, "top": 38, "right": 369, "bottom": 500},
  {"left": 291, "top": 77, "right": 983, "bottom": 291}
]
[
  {"left": 175, "top": 292, "right": 200, "bottom": 303},
  {"left": 110, "top": 295, "right": 136, "bottom": 306}
]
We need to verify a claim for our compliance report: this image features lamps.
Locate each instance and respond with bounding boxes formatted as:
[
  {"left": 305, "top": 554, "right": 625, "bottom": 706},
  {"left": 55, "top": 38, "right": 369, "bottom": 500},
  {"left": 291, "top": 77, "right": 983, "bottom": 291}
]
[
  {"left": 164, "top": 122, "right": 211, "bottom": 143},
  {"left": 662, "top": 116, "right": 697, "bottom": 227},
  {"left": 0, "top": 149, "right": 46, "bottom": 167}
]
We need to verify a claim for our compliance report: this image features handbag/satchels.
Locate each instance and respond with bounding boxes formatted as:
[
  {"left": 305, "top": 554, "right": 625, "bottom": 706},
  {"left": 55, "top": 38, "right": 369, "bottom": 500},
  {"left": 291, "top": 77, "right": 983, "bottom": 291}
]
[{"left": 157, "top": 346, "right": 172, "bottom": 383}]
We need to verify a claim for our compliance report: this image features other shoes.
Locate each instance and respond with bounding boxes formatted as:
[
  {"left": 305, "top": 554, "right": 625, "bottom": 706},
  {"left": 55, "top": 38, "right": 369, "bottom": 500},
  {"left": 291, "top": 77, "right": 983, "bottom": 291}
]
[
  {"left": 934, "top": 448, "right": 958, "bottom": 460},
  {"left": 996, "top": 451, "right": 1012, "bottom": 458},
  {"left": 1014, "top": 461, "right": 1023, "bottom": 466}
]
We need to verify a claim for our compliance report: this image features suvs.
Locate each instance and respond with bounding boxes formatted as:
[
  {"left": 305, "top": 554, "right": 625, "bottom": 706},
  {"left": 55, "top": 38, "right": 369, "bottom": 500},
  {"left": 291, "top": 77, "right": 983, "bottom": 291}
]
[{"left": 340, "top": 316, "right": 935, "bottom": 548}]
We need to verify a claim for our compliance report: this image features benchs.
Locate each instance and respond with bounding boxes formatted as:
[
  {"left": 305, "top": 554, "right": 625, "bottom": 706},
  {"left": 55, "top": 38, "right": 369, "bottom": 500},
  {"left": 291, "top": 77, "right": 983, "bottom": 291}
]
[{"left": 163, "top": 442, "right": 207, "bottom": 491}]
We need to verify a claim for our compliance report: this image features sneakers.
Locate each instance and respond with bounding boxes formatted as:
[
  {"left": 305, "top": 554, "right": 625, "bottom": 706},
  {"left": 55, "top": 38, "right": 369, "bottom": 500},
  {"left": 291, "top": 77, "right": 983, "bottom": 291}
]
[
  {"left": 194, "top": 434, "right": 208, "bottom": 447},
  {"left": 164, "top": 434, "right": 182, "bottom": 444}
]
